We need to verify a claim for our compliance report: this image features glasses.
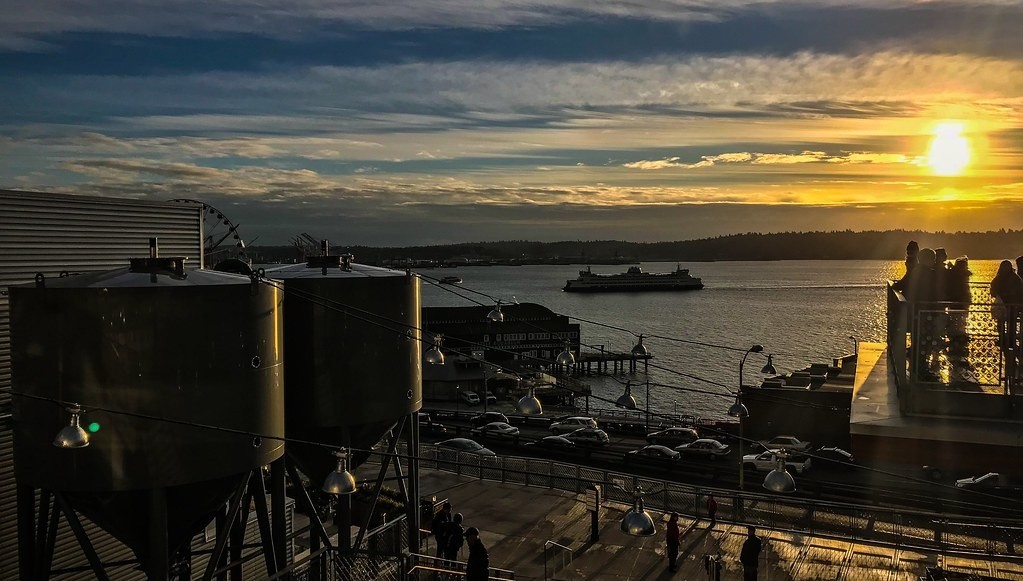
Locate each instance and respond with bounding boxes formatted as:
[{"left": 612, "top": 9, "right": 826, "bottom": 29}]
[{"left": 906, "top": 252, "right": 915, "bottom": 255}]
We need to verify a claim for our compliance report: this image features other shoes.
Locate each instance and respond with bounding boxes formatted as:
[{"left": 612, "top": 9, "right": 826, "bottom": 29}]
[
  {"left": 674, "top": 563, "right": 677, "bottom": 568},
  {"left": 669, "top": 568, "right": 677, "bottom": 573}
]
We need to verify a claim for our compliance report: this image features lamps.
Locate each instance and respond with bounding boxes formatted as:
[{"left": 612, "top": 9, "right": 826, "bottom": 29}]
[
  {"left": 52, "top": 401, "right": 91, "bottom": 449},
  {"left": 322, "top": 291, "right": 798, "bottom": 537}
]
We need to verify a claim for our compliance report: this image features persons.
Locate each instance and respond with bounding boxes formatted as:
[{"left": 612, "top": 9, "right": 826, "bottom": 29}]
[
  {"left": 891, "top": 241, "right": 971, "bottom": 382},
  {"left": 464, "top": 526, "right": 490, "bottom": 581},
  {"left": 990, "top": 256, "right": 1023, "bottom": 346},
  {"left": 431, "top": 503, "right": 464, "bottom": 577}
]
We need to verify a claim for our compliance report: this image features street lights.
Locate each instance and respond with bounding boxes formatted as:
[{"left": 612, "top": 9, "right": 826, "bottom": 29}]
[
  {"left": 849, "top": 334, "right": 858, "bottom": 356},
  {"left": 735, "top": 343, "right": 764, "bottom": 492}
]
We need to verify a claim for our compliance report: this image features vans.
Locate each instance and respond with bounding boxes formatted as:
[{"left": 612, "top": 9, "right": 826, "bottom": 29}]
[
  {"left": 461, "top": 390, "right": 480, "bottom": 405},
  {"left": 480, "top": 391, "right": 497, "bottom": 403}
]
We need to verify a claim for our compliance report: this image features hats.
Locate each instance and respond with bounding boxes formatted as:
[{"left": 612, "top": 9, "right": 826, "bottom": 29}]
[
  {"left": 748, "top": 526, "right": 756, "bottom": 531},
  {"left": 462, "top": 527, "right": 480, "bottom": 536},
  {"left": 907, "top": 240, "right": 919, "bottom": 251},
  {"left": 443, "top": 503, "right": 454, "bottom": 510}
]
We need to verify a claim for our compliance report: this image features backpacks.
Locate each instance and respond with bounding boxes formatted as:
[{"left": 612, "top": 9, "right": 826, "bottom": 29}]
[
  {"left": 431, "top": 512, "right": 445, "bottom": 534},
  {"left": 440, "top": 523, "right": 460, "bottom": 550}
]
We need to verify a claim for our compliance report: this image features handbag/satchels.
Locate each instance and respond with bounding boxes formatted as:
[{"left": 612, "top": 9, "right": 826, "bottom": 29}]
[{"left": 990, "top": 294, "right": 1009, "bottom": 321}]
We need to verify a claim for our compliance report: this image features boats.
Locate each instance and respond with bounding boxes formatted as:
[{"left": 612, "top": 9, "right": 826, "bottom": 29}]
[
  {"left": 440, "top": 275, "right": 462, "bottom": 285},
  {"left": 562, "top": 261, "right": 705, "bottom": 290}
]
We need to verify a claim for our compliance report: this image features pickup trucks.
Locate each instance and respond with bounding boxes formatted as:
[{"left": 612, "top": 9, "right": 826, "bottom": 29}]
[
  {"left": 955, "top": 472, "right": 1023, "bottom": 501},
  {"left": 737, "top": 448, "right": 813, "bottom": 477}
]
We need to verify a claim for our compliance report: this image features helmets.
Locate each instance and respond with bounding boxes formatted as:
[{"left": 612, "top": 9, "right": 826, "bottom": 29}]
[{"left": 453, "top": 513, "right": 463, "bottom": 524}]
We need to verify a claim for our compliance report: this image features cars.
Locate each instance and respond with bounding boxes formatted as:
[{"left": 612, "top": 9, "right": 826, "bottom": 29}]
[
  {"left": 646, "top": 426, "right": 698, "bottom": 444},
  {"left": 622, "top": 445, "right": 681, "bottom": 466},
  {"left": 469, "top": 422, "right": 521, "bottom": 438},
  {"left": 749, "top": 433, "right": 813, "bottom": 457},
  {"left": 674, "top": 438, "right": 731, "bottom": 461},
  {"left": 433, "top": 437, "right": 497, "bottom": 463},
  {"left": 558, "top": 426, "right": 610, "bottom": 447},
  {"left": 808, "top": 447, "right": 853, "bottom": 470},
  {"left": 549, "top": 416, "right": 599, "bottom": 436},
  {"left": 417, "top": 412, "right": 432, "bottom": 424},
  {"left": 420, "top": 423, "right": 447, "bottom": 433},
  {"left": 522, "top": 436, "right": 576, "bottom": 455},
  {"left": 468, "top": 411, "right": 509, "bottom": 428}
]
